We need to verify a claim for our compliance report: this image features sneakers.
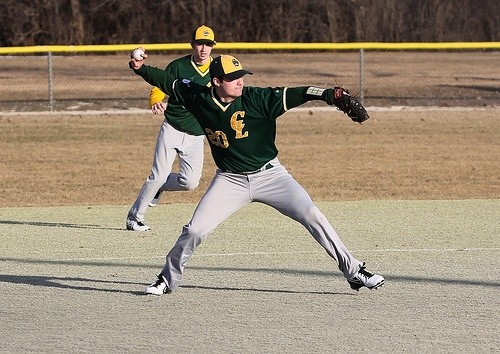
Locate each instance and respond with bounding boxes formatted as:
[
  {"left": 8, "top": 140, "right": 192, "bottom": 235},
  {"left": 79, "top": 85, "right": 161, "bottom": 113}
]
[
  {"left": 144, "top": 273, "right": 171, "bottom": 296},
  {"left": 346, "top": 261, "right": 386, "bottom": 292},
  {"left": 148, "top": 191, "right": 164, "bottom": 207},
  {"left": 126, "top": 219, "right": 151, "bottom": 231}
]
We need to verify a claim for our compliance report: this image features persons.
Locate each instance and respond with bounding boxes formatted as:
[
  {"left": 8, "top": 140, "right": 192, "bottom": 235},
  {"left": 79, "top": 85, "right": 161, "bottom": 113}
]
[
  {"left": 125, "top": 24, "right": 216, "bottom": 233},
  {"left": 127, "top": 46, "right": 385, "bottom": 296}
]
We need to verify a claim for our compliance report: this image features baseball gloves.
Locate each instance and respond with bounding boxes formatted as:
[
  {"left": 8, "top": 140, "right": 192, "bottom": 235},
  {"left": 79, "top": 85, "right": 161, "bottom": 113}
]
[{"left": 333, "top": 85, "right": 369, "bottom": 124}]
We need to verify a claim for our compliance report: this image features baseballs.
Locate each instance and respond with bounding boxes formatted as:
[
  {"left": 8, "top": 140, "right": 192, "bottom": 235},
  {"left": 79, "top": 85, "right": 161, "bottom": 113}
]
[{"left": 131, "top": 49, "right": 145, "bottom": 61}]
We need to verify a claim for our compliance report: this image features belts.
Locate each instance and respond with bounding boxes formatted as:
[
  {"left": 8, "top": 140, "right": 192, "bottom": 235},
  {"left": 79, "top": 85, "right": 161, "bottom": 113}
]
[{"left": 234, "top": 163, "right": 274, "bottom": 176}]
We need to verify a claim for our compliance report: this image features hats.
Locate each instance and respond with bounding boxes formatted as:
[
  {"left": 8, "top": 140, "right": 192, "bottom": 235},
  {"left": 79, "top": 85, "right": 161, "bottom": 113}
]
[
  {"left": 191, "top": 24, "right": 217, "bottom": 46},
  {"left": 209, "top": 54, "right": 253, "bottom": 81}
]
